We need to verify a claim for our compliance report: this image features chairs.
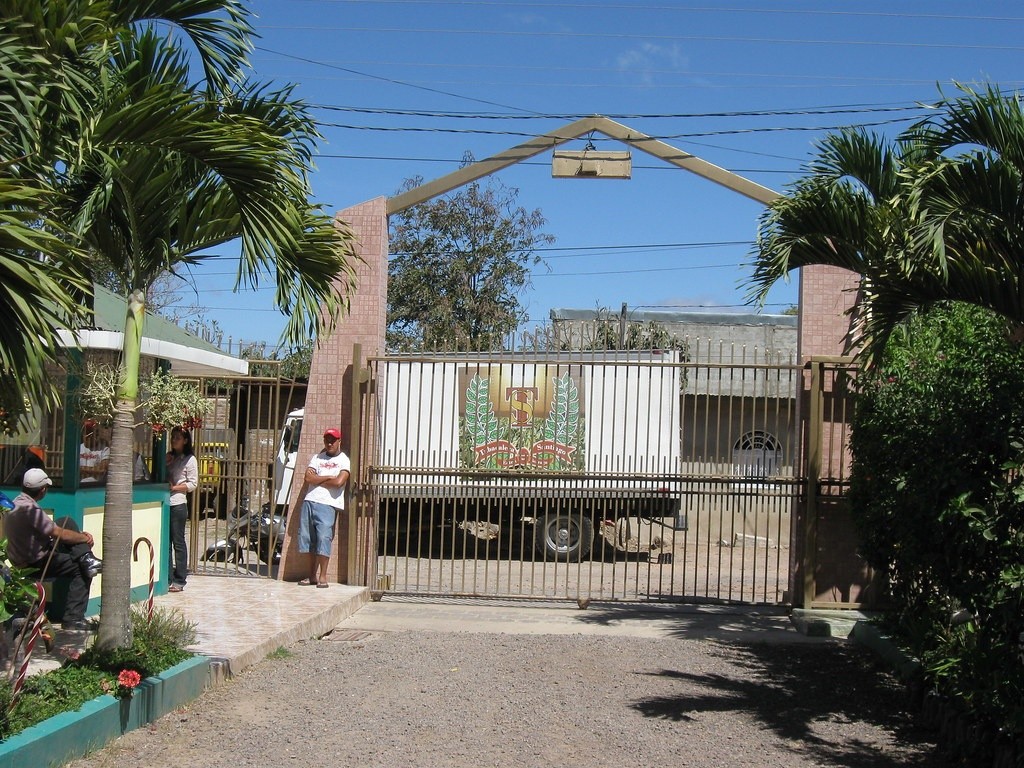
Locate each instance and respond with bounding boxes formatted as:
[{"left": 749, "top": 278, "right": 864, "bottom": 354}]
[{"left": 0, "top": 488, "right": 59, "bottom": 615}]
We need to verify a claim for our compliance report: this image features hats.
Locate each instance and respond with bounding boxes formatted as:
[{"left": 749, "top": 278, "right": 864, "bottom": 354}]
[
  {"left": 22, "top": 469, "right": 52, "bottom": 488},
  {"left": 85, "top": 418, "right": 97, "bottom": 429},
  {"left": 323, "top": 428, "right": 341, "bottom": 439}
]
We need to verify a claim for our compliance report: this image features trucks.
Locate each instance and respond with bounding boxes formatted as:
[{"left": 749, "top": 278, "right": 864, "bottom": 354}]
[
  {"left": 272, "top": 348, "right": 690, "bottom": 563},
  {"left": 143, "top": 442, "right": 228, "bottom": 518}
]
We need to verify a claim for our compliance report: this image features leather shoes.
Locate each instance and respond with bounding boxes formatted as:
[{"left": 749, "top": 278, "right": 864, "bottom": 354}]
[
  {"left": 80, "top": 553, "right": 102, "bottom": 577},
  {"left": 62, "top": 619, "right": 95, "bottom": 631}
]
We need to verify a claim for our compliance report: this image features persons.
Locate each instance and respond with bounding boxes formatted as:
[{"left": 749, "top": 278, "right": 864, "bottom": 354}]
[
  {"left": 4, "top": 467, "right": 102, "bottom": 630},
  {"left": 79, "top": 418, "right": 151, "bottom": 482},
  {"left": 297, "top": 427, "right": 350, "bottom": 587},
  {"left": 150, "top": 425, "right": 198, "bottom": 592}
]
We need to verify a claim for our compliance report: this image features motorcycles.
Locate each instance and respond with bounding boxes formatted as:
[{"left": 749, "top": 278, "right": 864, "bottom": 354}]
[{"left": 199, "top": 495, "right": 288, "bottom": 565}]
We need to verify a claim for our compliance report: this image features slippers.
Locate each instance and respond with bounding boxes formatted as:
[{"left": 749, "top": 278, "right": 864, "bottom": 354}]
[
  {"left": 316, "top": 581, "right": 329, "bottom": 588},
  {"left": 298, "top": 578, "right": 317, "bottom": 585}
]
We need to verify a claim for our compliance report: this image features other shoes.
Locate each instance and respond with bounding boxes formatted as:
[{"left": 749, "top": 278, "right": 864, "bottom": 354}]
[{"left": 168, "top": 585, "right": 183, "bottom": 592}]
